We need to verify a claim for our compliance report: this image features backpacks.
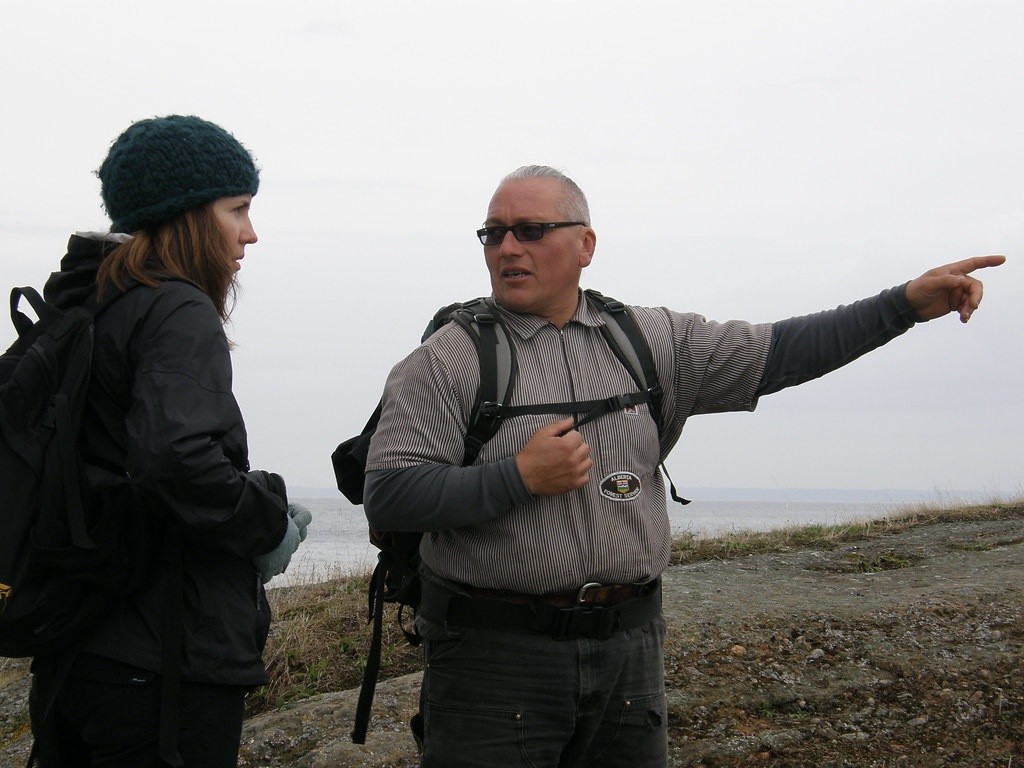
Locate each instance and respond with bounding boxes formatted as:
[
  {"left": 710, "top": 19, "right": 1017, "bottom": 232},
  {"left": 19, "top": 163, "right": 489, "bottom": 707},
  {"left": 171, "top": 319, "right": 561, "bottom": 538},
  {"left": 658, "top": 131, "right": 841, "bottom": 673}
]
[
  {"left": 0, "top": 270, "right": 210, "bottom": 658},
  {"left": 331, "top": 290, "right": 664, "bottom": 636}
]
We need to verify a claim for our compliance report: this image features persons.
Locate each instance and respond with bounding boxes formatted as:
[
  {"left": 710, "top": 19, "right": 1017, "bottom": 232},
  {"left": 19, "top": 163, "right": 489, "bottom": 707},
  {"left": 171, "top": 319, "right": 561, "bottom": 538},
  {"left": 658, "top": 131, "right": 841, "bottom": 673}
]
[
  {"left": 25, "top": 116, "right": 312, "bottom": 768},
  {"left": 359, "top": 165, "right": 1007, "bottom": 767}
]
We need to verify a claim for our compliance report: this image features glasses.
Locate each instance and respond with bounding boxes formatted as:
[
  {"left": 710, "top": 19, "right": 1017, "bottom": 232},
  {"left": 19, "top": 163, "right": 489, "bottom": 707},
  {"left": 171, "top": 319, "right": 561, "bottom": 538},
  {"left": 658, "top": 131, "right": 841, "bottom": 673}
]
[{"left": 478, "top": 222, "right": 587, "bottom": 245}]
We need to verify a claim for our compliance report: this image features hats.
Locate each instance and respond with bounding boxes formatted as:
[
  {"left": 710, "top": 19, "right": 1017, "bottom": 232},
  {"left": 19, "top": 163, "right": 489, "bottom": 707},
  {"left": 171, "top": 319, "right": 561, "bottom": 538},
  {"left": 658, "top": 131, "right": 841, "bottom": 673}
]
[{"left": 98, "top": 115, "right": 260, "bottom": 236}]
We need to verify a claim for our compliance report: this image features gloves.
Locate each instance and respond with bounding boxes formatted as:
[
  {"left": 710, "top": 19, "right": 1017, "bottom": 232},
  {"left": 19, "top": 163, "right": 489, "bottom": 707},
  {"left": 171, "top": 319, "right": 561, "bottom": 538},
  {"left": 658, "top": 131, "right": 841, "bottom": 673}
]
[
  {"left": 247, "top": 513, "right": 301, "bottom": 585},
  {"left": 287, "top": 503, "right": 312, "bottom": 542}
]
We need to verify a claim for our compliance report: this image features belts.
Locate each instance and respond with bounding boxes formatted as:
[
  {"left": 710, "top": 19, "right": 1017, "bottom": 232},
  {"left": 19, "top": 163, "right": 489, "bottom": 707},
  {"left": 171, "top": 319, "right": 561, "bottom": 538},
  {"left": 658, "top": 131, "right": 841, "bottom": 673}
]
[{"left": 417, "top": 560, "right": 662, "bottom": 616}]
